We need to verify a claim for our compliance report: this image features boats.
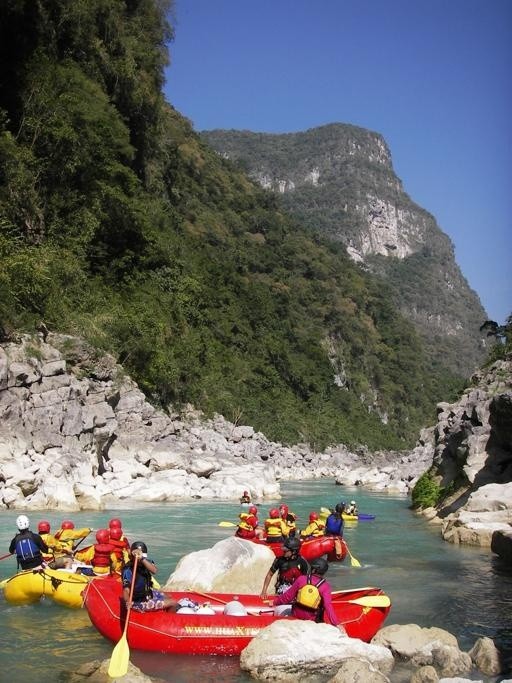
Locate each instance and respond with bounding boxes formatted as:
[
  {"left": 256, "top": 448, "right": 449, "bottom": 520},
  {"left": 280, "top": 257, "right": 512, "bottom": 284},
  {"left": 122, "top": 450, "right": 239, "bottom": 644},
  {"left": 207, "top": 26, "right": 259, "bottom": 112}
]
[
  {"left": 1, "top": 564, "right": 162, "bottom": 607},
  {"left": 235, "top": 527, "right": 347, "bottom": 560},
  {"left": 241, "top": 502, "right": 253, "bottom": 508},
  {"left": 80, "top": 574, "right": 391, "bottom": 655},
  {"left": 320, "top": 507, "right": 376, "bottom": 519}
]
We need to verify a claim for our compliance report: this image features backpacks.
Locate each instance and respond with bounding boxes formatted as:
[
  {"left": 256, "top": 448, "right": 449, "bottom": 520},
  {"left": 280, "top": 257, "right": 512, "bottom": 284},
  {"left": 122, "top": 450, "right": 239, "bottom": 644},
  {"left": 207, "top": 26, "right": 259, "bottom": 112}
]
[{"left": 296, "top": 575, "right": 325, "bottom": 610}]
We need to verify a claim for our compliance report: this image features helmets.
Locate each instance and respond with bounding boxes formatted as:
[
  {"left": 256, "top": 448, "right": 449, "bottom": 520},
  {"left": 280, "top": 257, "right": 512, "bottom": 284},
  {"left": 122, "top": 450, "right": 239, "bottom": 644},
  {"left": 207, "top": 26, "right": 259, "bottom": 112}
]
[
  {"left": 307, "top": 558, "right": 328, "bottom": 574},
  {"left": 109, "top": 527, "right": 123, "bottom": 539},
  {"left": 17, "top": 515, "right": 31, "bottom": 530},
  {"left": 108, "top": 519, "right": 122, "bottom": 528},
  {"left": 281, "top": 538, "right": 300, "bottom": 551},
  {"left": 249, "top": 508, "right": 257, "bottom": 514},
  {"left": 62, "top": 521, "right": 74, "bottom": 529},
  {"left": 97, "top": 529, "right": 109, "bottom": 544},
  {"left": 336, "top": 503, "right": 345, "bottom": 513},
  {"left": 130, "top": 542, "right": 147, "bottom": 558},
  {"left": 271, "top": 509, "right": 280, "bottom": 518},
  {"left": 38, "top": 521, "right": 50, "bottom": 533},
  {"left": 310, "top": 512, "right": 318, "bottom": 521},
  {"left": 278, "top": 505, "right": 288, "bottom": 515}
]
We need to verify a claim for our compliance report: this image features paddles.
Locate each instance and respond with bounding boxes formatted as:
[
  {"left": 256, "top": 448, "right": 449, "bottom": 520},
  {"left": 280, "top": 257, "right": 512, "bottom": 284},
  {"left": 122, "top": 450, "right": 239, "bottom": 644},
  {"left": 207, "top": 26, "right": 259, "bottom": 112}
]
[
  {"left": 342, "top": 539, "right": 361, "bottom": 567},
  {"left": 263, "top": 596, "right": 390, "bottom": 607},
  {"left": 218, "top": 521, "right": 237, "bottom": 527},
  {"left": 108, "top": 553, "right": 140, "bottom": 677}
]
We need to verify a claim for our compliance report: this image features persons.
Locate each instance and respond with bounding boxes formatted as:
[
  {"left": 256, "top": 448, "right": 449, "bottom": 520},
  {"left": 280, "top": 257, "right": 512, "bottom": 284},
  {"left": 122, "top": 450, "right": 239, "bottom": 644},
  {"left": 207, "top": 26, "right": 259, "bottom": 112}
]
[
  {"left": 325, "top": 503, "right": 344, "bottom": 538},
  {"left": 36, "top": 521, "right": 72, "bottom": 570},
  {"left": 298, "top": 512, "right": 325, "bottom": 542},
  {"left": 109, "top": 520, "right": 123, "bottom": 528},
  {"left": 54, "top": 520, "right": 94, "bottom": 550},
  {"left": 9, "top": 515, "right": 47, "bottom": 569},
  {"left": 261, "top": 538, "right": 311, "bottom": 599},
  {"left": 78, "top": 529, "right": 116, "bottom": 575},
  {"left": 279, "top": 505, "right": 296, "bottom": 538},
  {"left": 123, "top": 542, "right": 156, "bottom": 609},
  {"left": 264, "top": 509, "right": 288, "bottom": 541},
  {"left": 241, "top": 491, "right": 252, "bottom": 506},
  {"left": 346, "top": 501, "right": 358, "bottom": 515},
  {"left": 272, "top": 558, "right": 346, "bottom": 633},
  {"left": 236, "top": 507, "right": 264, "bottom": 541},
  {"left": 108, "top": 528, "right": 128, "bottom": 571}
]
